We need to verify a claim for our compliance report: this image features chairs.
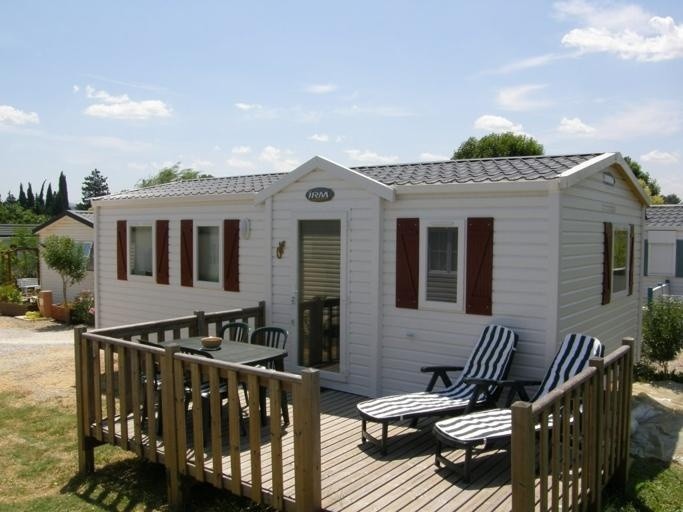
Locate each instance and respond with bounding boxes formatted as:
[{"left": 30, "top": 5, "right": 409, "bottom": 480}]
[
  {"left": 433, "top": 333, "right": 605, "bottom": 482},
  {"left": 251, "top": 327, "right": 288, "bottom": 426},
  {"left": 218, "top": 323, "right": 249, "bottom": 434},
  {"left": 136, "top": 339, "right": 190, "bottom": 436},
  {"left": 357, "top": 324, "right": 519, "bottom": 456},
  {"left": 177, "top": 347, "right": 246, "bottom": 446}
]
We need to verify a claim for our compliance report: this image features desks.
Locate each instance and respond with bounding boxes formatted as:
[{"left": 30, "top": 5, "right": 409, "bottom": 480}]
[{"left": 158, "top": 336, "right": 288, "bottom": 436}]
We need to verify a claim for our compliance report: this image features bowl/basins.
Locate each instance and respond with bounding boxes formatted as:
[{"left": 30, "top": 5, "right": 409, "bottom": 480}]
[{"left": 200, "top": 336, "right": 222, "bottom": 348}]
